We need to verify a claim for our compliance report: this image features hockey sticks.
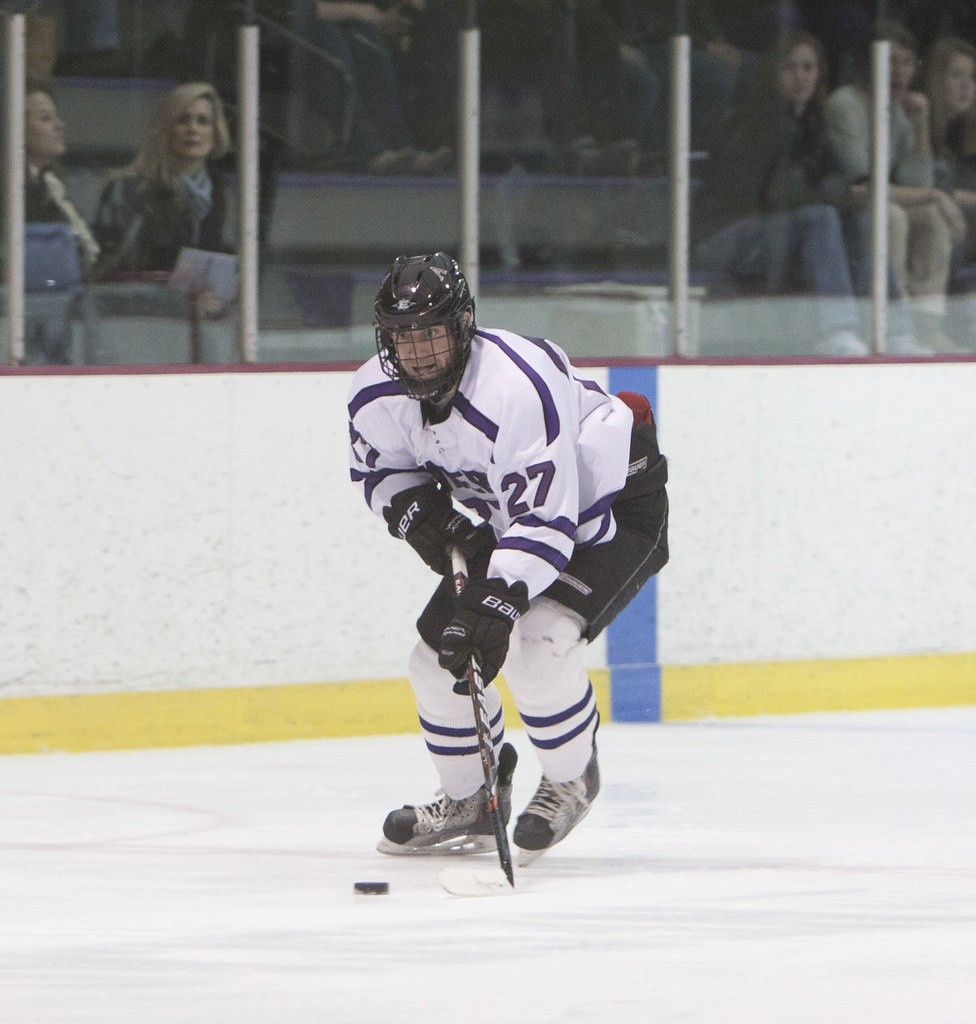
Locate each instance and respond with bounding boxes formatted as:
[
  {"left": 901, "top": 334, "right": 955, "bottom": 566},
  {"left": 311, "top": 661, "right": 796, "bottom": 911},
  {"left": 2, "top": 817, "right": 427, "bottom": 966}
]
[{"left": 438, "top": 546, "right": 515, "bottom": 897}]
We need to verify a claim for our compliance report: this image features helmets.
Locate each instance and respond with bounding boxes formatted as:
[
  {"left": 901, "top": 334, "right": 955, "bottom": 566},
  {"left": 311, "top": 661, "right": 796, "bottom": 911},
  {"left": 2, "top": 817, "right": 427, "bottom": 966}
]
[{"left": 373, "top": 250, "right": 477, "bottom": 402}]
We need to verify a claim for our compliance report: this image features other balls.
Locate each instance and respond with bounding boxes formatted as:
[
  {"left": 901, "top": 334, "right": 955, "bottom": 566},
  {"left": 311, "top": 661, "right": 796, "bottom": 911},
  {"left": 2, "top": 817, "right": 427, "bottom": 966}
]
[{"left": 353, "top": 881, "right": 390, "bottom": 895}]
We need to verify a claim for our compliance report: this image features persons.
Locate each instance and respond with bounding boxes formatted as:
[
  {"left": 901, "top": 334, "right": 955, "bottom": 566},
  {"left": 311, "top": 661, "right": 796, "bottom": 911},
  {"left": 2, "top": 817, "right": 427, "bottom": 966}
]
[
  {"left": 346, "top": 250, "right": 672, "bottom": 866},
  {"left": 89, "top": 82, "right": 232, "bottom": 364},
  {"left": 688, "top": 34, "right": 933, "bottom": 358},
  {"left": 818, "top": 16, "right": 970, "bottom": 352},
  {"left": 916, "top": 38, "right": 975, "bottom": 227},
  {"left": 222, "top": 0, "right": 968, "bottom": 172},
  {"left": 24, "top": 80, "right": 112, "bottom": 365}
]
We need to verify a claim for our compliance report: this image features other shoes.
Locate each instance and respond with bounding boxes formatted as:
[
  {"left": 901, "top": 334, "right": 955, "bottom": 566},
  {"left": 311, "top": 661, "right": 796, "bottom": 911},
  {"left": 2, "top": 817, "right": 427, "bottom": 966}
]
[
  {"left": 880, "top": 321, "right": 974, "bottom": 356},
  {"left": 811, "top": 324, "right": 872, "bottom": 356}
]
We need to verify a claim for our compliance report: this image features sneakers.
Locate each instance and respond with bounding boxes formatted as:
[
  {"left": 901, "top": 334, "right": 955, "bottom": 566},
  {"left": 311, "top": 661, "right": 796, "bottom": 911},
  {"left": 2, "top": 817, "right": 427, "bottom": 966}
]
[
  {"left": 512, "top": 746, "right": 602, "bottom": 867},
  {"left": 377, "top": 743, "right": 519, "bottom": 857}
]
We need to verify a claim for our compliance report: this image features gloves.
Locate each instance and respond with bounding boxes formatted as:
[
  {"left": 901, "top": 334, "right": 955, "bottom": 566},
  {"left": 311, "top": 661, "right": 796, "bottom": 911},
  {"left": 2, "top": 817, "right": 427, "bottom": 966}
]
[
  {"left": 438, "top": 577, "right": 530, "bottom": 696},
  {"left": 381, "top": 483, "right": 480, "bottom": 576}
]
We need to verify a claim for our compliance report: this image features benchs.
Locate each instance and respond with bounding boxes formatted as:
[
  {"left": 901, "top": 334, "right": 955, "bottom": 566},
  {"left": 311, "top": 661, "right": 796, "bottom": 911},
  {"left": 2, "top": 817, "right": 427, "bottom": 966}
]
[
  {"left": 220, "top": 174, "right": 697, "bottom": 253},
  {"left": 351, "top": 268, "right": 976, "bottom": 357},
  {"left": 30, "top": 77, "right": 180, "bottom": 153}
]
